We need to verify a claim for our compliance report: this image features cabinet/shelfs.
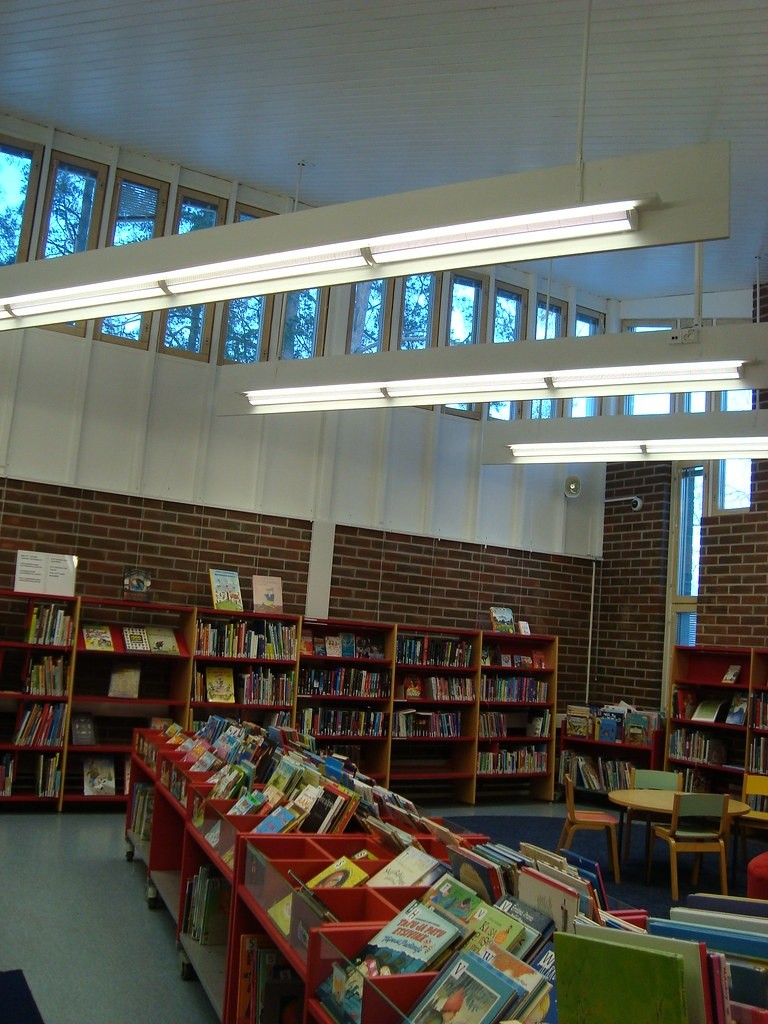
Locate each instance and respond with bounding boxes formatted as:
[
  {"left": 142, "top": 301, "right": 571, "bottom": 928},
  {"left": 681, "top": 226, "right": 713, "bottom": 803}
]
[{"left": 0, "top": 586, "right": 768, "bottom": 1024}]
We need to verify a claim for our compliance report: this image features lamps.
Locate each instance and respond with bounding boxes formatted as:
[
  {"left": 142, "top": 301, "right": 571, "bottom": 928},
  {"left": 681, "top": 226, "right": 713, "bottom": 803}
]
[{"left": 0, "top": 0, "right": 768, "bottom": 467}]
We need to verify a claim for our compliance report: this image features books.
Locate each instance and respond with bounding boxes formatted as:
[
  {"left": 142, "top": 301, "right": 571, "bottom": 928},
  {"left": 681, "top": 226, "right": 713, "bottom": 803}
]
[{"left": 0, "top": 569, "right": 768, "bottom": 1024}]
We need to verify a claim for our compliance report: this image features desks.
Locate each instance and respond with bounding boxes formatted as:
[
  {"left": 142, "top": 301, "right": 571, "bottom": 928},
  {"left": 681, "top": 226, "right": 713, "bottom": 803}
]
[{"left": 606, "top": 789, "right": 752, "bottom": 887}]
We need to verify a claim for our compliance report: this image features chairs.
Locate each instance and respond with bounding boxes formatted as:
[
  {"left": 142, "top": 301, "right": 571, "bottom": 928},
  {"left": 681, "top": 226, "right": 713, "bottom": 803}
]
[
  {"left": 623, "top": 767, "right": 683, "bottom": 867},
  {"left": 556, "top": 773, "right": 621, "bottom": 883},
  {"left": 648, "top": 792, "right": 730, "bottom": 901},
  {"left": 730, "top": 773, "right": 768, "bottom": 869}
]
